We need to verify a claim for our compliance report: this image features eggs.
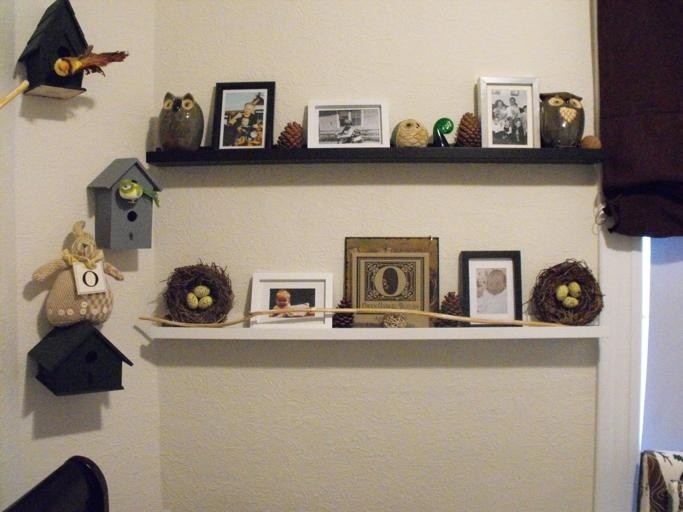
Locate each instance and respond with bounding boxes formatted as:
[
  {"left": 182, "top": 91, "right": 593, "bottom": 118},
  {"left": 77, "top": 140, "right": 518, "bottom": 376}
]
[
  {"left": 186, "top": 293, "right": 198, "bottom": 310},
  {"left": 568, "top": 281, "right": 581, "bottom": 297},
  {"left": 563, "top": 296, "right": 579, "bottom": 309},
  {"left": 198, "top": 296, "right": 213, "bottom": 310},
  {"left": 556, "top": 284, "right": 568, "bottom": 301},
  {"left": 194, "top": 285, "right": 211, "bottom": 298}
]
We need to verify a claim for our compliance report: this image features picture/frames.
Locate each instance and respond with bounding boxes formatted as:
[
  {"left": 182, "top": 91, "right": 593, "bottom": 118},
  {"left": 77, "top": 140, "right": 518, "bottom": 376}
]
[
  {"left": 249, "top": 272, "right": 333, "bottom": 329},
  {"left": 479, "top": 77, "right": 541, "bottom": 148},
  {"left": 211, "top": 81, "right": 275, "bottom": 150},
  {"left": 307, "top": 100, "right": 390, "bottom": 149},
  {"left": 461, "top": 248, "right": 522, "bottom": 327}
]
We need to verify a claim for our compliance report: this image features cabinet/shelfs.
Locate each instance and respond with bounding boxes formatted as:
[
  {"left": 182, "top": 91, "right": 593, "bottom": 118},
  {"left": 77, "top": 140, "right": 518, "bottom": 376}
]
[{"left": 135, "top": 146, "right": 611, "bottom": 342}]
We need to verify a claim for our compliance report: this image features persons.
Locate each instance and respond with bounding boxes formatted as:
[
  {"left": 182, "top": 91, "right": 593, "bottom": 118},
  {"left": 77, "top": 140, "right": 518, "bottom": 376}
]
[
  {"left": 231, "top": 102, "right": 256, "bottom": 145},
  {"left": 477, "top": 270, "right": 506, "bottom": 313},
  {"left": 493, "top": 96, "right": 525, "bottom": 143},
  {"left": 333, "top": 117, "right": 365, "bottom": 144},
  {"left": 269, "top": 291, "right": 314, "bottom": 317}
]
